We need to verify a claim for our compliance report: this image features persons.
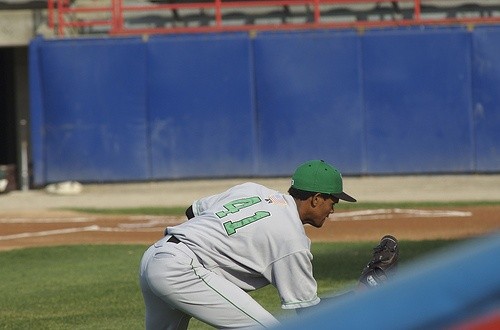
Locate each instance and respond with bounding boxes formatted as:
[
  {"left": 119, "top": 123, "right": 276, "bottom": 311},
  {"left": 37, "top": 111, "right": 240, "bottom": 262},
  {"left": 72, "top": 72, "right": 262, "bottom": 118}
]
[{"left": 140, "top": 159, "right": 399, "bottom": 330}]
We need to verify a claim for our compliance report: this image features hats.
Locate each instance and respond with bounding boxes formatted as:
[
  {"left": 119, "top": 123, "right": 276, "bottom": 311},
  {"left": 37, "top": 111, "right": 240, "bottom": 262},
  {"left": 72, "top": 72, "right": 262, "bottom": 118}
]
[{"left": 291, "top": 159, "right": 357, "bottom": 202}]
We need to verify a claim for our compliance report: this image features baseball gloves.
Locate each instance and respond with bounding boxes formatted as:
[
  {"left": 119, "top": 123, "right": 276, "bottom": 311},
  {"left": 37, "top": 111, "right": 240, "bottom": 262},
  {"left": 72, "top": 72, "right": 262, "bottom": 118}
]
[{"left": 357, "top": 234, "right": 400, "bottom": 291}]
